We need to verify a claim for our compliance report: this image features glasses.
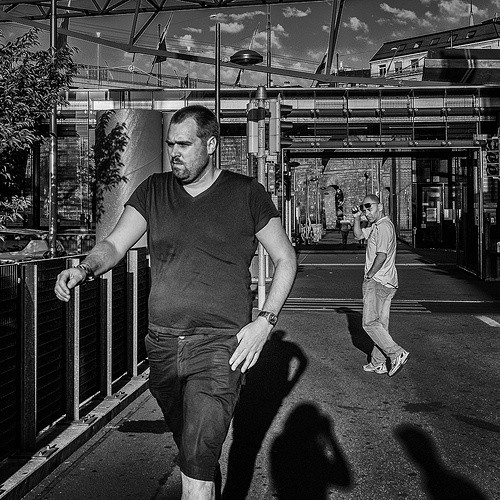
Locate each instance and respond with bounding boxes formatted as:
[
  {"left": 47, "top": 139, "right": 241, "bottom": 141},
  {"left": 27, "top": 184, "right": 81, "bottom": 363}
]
[{"left": 356, "top": 202, "right": 378, "bottom": 211}]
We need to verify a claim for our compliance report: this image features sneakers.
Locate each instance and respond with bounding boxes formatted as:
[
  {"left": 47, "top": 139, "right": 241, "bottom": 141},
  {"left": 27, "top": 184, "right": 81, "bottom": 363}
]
[
  {"left": 362, "top": 362, "right": 388, "bottom": 374},
  {"left": 388, "top": 351, "right": 411, "bottom": 376}
]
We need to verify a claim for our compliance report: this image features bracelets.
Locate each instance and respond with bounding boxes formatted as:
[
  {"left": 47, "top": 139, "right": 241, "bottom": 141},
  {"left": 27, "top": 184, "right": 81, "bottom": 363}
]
[
  {"left": 73, "top": 265, "right": 90, "bottom": 286},
  {"left": 79, "top": 263, "right": 95, "bottom": 281}
]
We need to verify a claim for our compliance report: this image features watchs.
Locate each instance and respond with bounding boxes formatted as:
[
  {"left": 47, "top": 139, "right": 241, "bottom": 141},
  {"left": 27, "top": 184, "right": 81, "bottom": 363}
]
[{"left": 257, "top": 311, "right": 278, "bottom": 327}]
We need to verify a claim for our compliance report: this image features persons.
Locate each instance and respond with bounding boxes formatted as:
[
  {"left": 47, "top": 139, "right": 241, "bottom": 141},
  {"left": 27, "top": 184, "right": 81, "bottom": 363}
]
[
  {"left": 54, "top": 106, "right": 297, "bottom": 500},
  {"left": 351, "top": 195, "right": 411, "bottom": 377},
  {"left": 336, "top": 215, "right": 364, "bottom": 248}
]
